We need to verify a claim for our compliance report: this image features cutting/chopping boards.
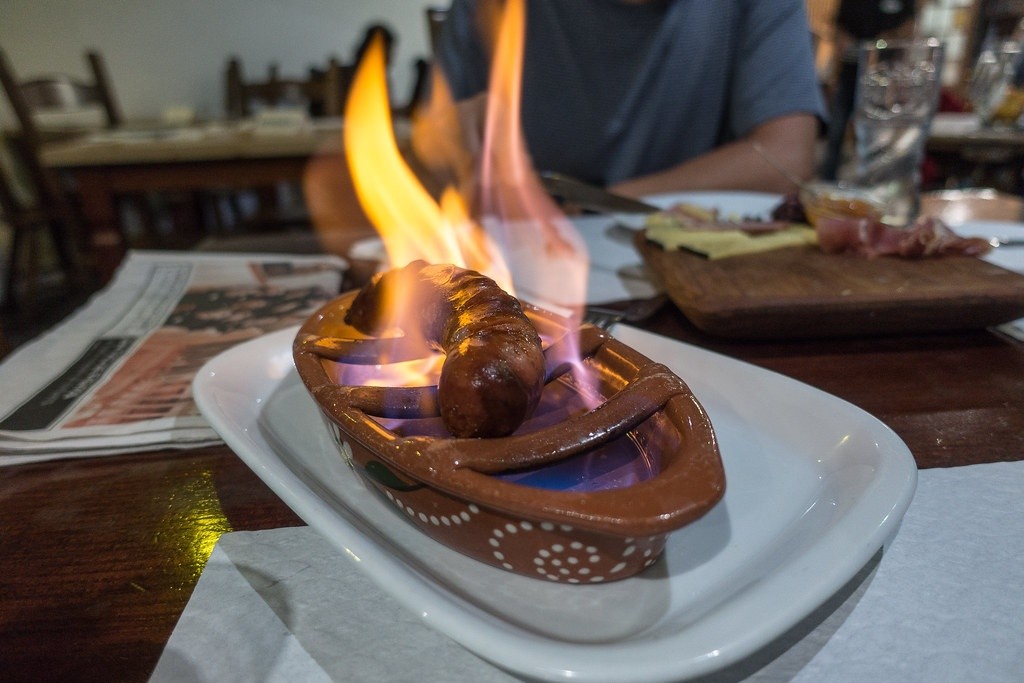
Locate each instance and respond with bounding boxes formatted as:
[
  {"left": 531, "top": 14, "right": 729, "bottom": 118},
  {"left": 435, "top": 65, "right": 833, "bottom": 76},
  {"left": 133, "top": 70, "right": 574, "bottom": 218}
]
[{"left": 637, "top": 225, "right": 1024, "bottom": 337}]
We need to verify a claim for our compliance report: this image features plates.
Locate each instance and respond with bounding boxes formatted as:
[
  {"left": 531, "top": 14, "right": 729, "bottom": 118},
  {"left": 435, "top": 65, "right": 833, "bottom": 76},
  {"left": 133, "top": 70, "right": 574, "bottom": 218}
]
[
  {"left": 190, "top": 322, "right": 919, "bottom": 683},
  {"left": 348, "top": 216, "right": 661, "bottom": 308},
  {"left": 291, "top": 285, "right": 728, "bottom": 585},
  {"left": 623, "top": 192, "right": 789, "bottom": 222}
]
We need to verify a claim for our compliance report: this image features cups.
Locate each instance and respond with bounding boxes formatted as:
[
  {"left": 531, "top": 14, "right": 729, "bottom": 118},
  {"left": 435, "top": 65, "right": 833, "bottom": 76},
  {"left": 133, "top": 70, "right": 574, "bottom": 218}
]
[{"left": 836, "top": 37, "right": 944, "bottom": 223}]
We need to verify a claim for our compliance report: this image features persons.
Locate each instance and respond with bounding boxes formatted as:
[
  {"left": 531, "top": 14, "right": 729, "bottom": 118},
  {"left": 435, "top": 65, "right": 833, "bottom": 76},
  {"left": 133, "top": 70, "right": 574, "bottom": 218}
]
[
  {"left": 438, "top": 0, "right": 828, "bottom": 196},
  {"left": 339, "top": 23, "right": 397, "bottom": 116},
  {"left": 832, "top": 0, "right": 922, "bottom": 153},
  {"left": 190, "top": 288, "right": 326, "bottom": 327}
]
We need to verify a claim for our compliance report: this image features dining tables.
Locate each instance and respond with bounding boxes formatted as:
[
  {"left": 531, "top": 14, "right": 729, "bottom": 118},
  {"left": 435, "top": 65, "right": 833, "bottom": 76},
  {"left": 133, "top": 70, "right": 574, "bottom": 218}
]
[{"left": 0, "top": 229, "right": 1024, "bottom": 683}]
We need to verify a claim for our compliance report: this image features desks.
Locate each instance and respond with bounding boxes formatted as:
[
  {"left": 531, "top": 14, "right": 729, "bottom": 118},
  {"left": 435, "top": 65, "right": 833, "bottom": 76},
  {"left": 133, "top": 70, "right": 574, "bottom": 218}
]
[{"left": 46, "top": 114, "right": 412, "bottom": 291}]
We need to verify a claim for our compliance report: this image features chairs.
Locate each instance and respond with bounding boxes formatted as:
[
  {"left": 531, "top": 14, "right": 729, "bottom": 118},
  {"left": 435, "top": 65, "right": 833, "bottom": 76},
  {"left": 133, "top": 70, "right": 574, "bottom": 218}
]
[{"left": 0, "top": 46, "right": 352, "bottom": 358}]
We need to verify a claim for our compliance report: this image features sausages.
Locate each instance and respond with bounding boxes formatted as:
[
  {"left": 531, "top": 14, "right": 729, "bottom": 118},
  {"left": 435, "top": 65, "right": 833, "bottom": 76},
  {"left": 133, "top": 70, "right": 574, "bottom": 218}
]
[{"left": 343, "top": 261, "right": 548, "bottom": 439}]
